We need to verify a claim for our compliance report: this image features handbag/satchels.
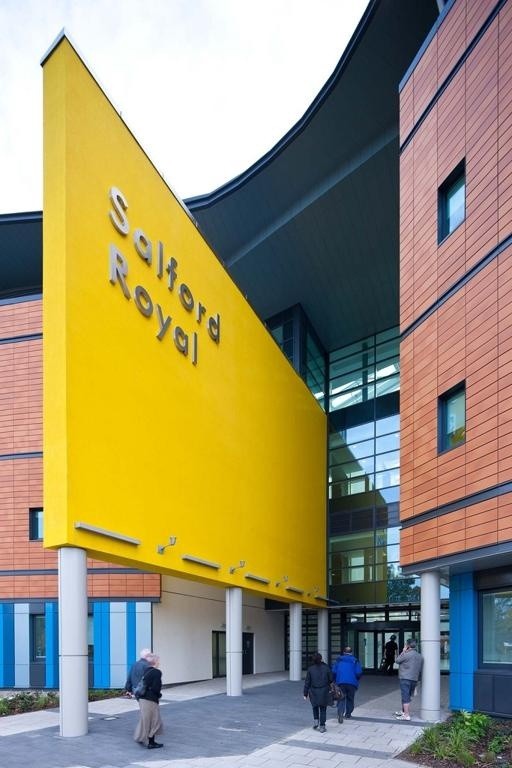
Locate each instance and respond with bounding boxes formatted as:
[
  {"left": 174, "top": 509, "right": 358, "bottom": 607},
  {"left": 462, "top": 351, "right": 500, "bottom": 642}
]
[
  {"left": 134, "top": 667, "right": 154, "bottom": 699},
  {"left": 329, "top": 682, "right": 344, "bottom": 700}
]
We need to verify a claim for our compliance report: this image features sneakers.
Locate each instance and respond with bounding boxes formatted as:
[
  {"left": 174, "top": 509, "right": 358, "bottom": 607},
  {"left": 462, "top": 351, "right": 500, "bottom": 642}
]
[
  {"left": 320, "top": 726, "right": 327, "bottom": 732},
  {"left": 396, "top": 714, "right": 411, "bottom": 720},
  {"left": 396, "top": 710, "right": 404, "bottom": 715},
  {"left": 313, "top": 719, "right": 319, "bottom": 729}
]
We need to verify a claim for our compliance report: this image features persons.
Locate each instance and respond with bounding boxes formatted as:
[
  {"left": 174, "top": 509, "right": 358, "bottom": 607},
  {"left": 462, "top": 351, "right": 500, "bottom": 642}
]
[
  {"left": 394, "top": 638, "right": 423, "bottom": 721},
  {"left": 381, "top": 634, "right": 398, "bottom": 675},
  {"left": 303, "top": 652, "right": 334, "bottom": 733},
  {"left": 123, "top": 648, "right": 152, "bottom": 745},
  {"left": 135, "top": 652, "right": 164, "bottom": 749},
  {"left": 329, "top": 646, "right": 363, "bottom": 723}
]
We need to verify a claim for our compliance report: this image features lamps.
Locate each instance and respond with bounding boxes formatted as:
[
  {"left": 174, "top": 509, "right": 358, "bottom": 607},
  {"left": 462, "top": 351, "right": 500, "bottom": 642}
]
[{"left": 76, "top": 518, "right": 341, "bottom": 609}]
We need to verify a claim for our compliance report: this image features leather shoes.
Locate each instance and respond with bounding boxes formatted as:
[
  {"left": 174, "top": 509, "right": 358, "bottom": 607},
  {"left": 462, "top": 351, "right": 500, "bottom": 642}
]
[
  {"left": 346, "top": 713, "right": 351, "bottom": 717},
  {"left": 339, "top": 717, "right": 343, "bottom": 722},
  {"left": 147, "top": 742, "right": 163, "bottom": 748}
]
